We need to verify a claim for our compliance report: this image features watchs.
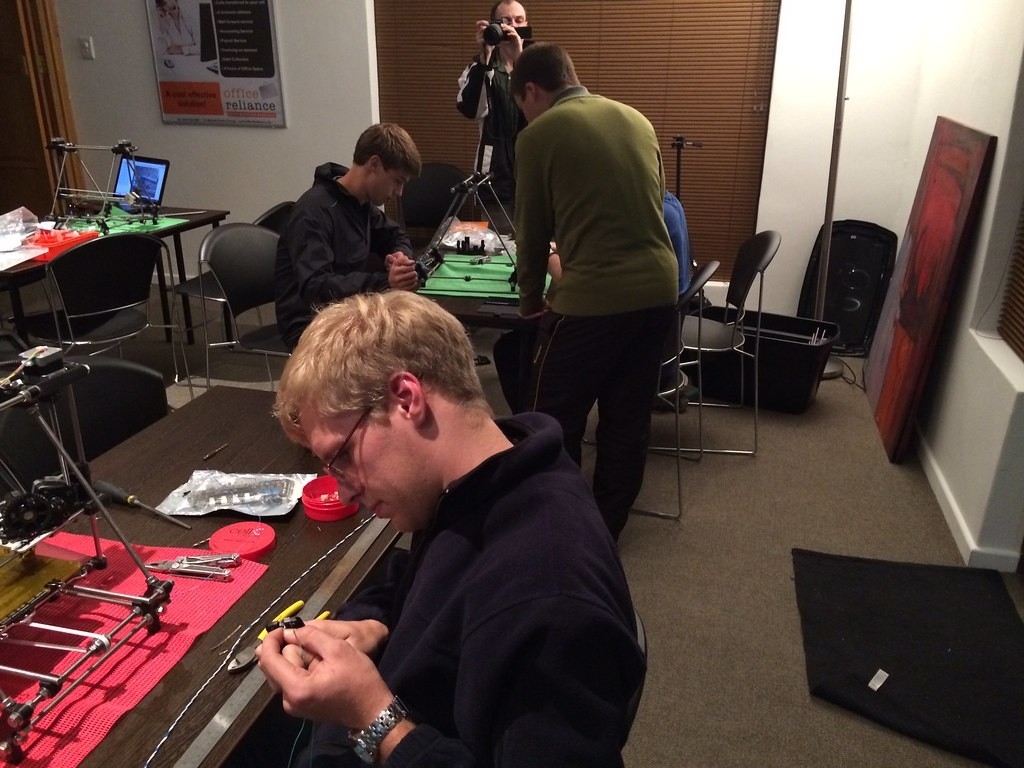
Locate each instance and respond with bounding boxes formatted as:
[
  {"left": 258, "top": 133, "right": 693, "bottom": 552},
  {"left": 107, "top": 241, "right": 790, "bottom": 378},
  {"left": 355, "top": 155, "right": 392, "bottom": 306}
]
[{"left": 347, "top": 694, "right": 410, "bottom": 764}]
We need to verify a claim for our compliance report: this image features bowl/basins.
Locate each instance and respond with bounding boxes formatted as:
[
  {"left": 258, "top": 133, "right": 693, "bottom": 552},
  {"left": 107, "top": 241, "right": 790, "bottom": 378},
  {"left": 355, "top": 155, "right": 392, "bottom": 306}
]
[{"left": 302, "top": 475, "right": 361, "bottom": 521}]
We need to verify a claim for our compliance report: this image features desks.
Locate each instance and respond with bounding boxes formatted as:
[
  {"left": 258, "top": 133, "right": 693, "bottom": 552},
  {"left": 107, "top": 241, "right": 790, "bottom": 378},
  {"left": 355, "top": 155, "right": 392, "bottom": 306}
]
[
  {"left": 0, "top": 203, "right": 230, "bottom": 357},
  {"left": 408, "top": 234, "right": 552, "bottom": 332},
  {"left": 0, "top": 384, "right": 404, "bottom": 768}
]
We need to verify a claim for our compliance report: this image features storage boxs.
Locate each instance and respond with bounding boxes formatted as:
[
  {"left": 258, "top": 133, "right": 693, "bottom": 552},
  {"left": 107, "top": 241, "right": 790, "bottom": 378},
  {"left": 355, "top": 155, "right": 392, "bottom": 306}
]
[{"left": 688, "top": 305, "right": 841, "bottom": 412}]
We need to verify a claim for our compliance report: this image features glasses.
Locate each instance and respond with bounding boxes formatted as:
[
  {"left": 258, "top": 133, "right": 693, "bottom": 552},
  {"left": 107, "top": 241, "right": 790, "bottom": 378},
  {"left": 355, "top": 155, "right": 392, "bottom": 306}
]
[{"left": 324, "top": 404, "right": 377, "bottom": 481}]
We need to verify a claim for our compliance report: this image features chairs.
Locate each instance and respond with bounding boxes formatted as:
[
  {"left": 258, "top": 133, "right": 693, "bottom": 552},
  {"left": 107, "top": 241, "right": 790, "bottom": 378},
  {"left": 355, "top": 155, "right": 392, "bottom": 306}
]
[
  {"left": 645, "top": 230, "right": 780, "bottom": 453},
  {"left": 626, "top": 258, "right": 720, "bottom": 521},
  {"left": 0, "top": 160, "right": 464, "bottom": 497}
]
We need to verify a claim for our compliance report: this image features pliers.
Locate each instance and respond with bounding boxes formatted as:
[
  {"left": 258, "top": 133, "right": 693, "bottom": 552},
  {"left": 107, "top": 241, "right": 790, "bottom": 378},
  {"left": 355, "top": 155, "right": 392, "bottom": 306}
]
[
  {"left": 227, "top": 600, "right": 331, "bottom": 672},
  {"left": 266, "top": 616, "right": 305, "bottom": 633},
  {"left": 145, "top": 553, "right": 242, "bottom": 582}
]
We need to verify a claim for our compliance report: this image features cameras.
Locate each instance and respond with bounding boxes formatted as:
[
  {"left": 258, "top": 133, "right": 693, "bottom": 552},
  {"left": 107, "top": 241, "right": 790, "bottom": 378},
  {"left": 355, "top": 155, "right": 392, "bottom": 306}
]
[{"left": 483, "top": 21, "right": 532, "bottom": 45}]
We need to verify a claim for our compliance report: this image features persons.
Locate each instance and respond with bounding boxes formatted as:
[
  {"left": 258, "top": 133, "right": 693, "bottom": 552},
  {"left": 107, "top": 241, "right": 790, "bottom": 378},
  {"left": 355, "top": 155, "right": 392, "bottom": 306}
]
[
  {"left": 510, "top": 39, "right": 679, "bottom": 546},
  {"left": 253, "top": 284, "right": 646, "bottom": 768},
  {"left": 156, "top": 0, "right": 201, "bottom": 60},
  {"left": 492, "top": 186, "right": 692, "bottom": 415},
  {"left": 458, "top": 3, "right": 539, "bottom": 236},
  {"left": 276, "top": 122, "right": 421, "bottom": 355}
]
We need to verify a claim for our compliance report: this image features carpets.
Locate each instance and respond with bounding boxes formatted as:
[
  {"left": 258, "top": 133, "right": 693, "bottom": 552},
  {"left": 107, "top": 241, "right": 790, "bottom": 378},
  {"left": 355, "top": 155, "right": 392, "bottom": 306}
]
[{"left": 792, "top": 547, "right": 1024, "bottom": 768}]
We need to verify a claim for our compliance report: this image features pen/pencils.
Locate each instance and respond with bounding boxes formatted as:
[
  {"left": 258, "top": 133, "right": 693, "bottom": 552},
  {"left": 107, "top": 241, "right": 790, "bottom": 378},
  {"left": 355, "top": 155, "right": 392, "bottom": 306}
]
[{"left": 203, "top": 444, "right": 228, "bottom": 459}]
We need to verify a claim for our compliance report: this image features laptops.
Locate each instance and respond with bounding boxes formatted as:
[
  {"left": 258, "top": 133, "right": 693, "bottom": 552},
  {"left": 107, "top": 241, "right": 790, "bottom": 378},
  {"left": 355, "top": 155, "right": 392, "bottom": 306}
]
[{"left": 113, "top": 154, "right": 169, "bottom": 214}]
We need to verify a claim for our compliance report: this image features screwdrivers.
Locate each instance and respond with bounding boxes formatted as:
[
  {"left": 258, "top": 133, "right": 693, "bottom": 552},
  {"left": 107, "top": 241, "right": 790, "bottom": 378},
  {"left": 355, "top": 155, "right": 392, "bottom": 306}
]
[{"left": 94, "top": 480, "right": 192, "bottom": 530}]
